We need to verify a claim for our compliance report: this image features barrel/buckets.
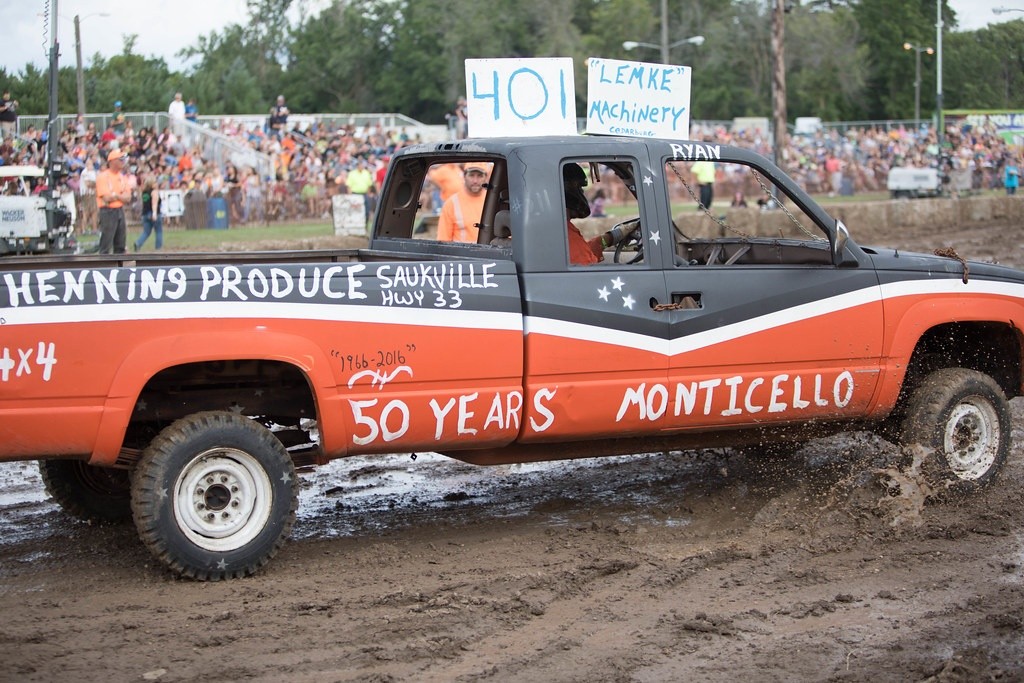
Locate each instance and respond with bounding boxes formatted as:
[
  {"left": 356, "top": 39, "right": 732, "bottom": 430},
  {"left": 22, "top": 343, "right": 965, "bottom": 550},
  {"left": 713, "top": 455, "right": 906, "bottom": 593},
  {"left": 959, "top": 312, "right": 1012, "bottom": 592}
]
[{"left": 206, "top": 199, "right": 228, "bottom": 230}]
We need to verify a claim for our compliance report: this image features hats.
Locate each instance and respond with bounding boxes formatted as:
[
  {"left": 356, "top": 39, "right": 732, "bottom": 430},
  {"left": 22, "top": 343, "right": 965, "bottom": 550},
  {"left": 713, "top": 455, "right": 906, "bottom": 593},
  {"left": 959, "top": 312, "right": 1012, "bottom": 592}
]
[
  {"left": 108, "top": 148, "right": 125, "bottom": 162},
  {"left": 464, "top": 160, "right": 486, "bottom": 175}
]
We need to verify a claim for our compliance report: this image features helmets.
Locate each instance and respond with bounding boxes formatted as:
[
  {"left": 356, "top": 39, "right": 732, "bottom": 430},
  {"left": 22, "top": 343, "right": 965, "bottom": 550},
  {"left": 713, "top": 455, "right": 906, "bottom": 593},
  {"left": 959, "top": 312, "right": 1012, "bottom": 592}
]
[{"left": 562, "top": 163, "right": 590, "bottom": 219}]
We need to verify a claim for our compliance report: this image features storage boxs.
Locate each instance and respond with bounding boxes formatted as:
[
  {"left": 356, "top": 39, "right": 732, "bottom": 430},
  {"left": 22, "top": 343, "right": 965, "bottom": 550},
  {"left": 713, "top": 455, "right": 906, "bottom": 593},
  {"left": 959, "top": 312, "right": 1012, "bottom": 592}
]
[{"left": 185, "top": 190, "right": 227, "bottom": 229}]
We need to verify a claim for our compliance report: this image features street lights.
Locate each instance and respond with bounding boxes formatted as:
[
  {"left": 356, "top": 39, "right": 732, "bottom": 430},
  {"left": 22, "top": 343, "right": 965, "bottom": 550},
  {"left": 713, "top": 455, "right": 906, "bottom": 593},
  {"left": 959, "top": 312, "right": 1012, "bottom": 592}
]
[
  {"left": 36, "top": 11, "right": 111, "bottom": 114},
  {"left": 623, "top": 36, "right": 706, "bottom": 64},
  {"left": 903, "top": 42, "right": 934, "bottom": 133}
]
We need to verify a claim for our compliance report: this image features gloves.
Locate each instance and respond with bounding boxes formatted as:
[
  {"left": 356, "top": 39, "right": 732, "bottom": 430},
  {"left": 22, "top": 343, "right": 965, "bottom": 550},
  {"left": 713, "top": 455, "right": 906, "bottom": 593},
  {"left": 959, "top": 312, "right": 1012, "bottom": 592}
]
[{"left": 605, "top": 220, "right": 643, "bottom": 247}]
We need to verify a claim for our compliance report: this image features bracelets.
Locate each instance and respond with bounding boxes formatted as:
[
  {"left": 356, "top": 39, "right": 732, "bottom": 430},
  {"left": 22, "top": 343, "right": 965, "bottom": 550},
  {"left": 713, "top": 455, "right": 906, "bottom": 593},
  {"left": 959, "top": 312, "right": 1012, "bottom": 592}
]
[{"left": 598, "top": 236, "right": 608, "bottom": 249}]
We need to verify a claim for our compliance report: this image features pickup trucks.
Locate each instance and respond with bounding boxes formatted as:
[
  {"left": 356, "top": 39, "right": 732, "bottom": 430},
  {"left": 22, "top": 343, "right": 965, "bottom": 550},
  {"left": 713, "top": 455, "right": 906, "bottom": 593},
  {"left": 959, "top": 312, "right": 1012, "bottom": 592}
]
[
  {"left": 0, "top": 137, "right": 1024, "bottom": 580},
  {"left": 0, "top": 166, "right": 77, "bottom": 257}
]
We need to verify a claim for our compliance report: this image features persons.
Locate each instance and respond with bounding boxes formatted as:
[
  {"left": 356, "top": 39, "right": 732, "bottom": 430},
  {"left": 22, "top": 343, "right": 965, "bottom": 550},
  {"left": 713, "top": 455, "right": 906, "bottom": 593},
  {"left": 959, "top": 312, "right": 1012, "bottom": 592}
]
[
  {"left": 0, "top": 91, "right": 490, "bottom": 261},
  {"left": 562, "top": 164, "right": 642, "bottom": 265},
  {"left": 662, "top": 114, "right": 1024, "bottom": 212}
]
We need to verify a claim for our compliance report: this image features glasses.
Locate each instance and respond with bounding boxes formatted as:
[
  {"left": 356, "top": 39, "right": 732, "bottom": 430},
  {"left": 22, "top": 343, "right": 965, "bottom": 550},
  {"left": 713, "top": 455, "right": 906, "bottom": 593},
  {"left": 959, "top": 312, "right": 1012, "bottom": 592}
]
[{"left": 117, "top": 157, "right": 125, "bottom": 161}]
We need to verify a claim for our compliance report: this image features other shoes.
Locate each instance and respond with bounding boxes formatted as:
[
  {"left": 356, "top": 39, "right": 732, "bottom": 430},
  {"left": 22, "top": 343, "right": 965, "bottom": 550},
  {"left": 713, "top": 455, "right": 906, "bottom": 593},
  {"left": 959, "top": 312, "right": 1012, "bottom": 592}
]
[{"left": 134, "top": 241, "right": 140, "bottom": 252}]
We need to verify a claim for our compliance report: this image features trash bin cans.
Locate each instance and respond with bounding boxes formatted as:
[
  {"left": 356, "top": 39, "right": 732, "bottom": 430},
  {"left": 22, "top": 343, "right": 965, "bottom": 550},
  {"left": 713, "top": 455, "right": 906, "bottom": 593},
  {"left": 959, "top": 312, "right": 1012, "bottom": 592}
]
[
  {"left": 183, "top": 190, "right": 209, "bottom": 230},
  {"left": 206, "top": 197, "right": 229, "bottom": 229}
]
